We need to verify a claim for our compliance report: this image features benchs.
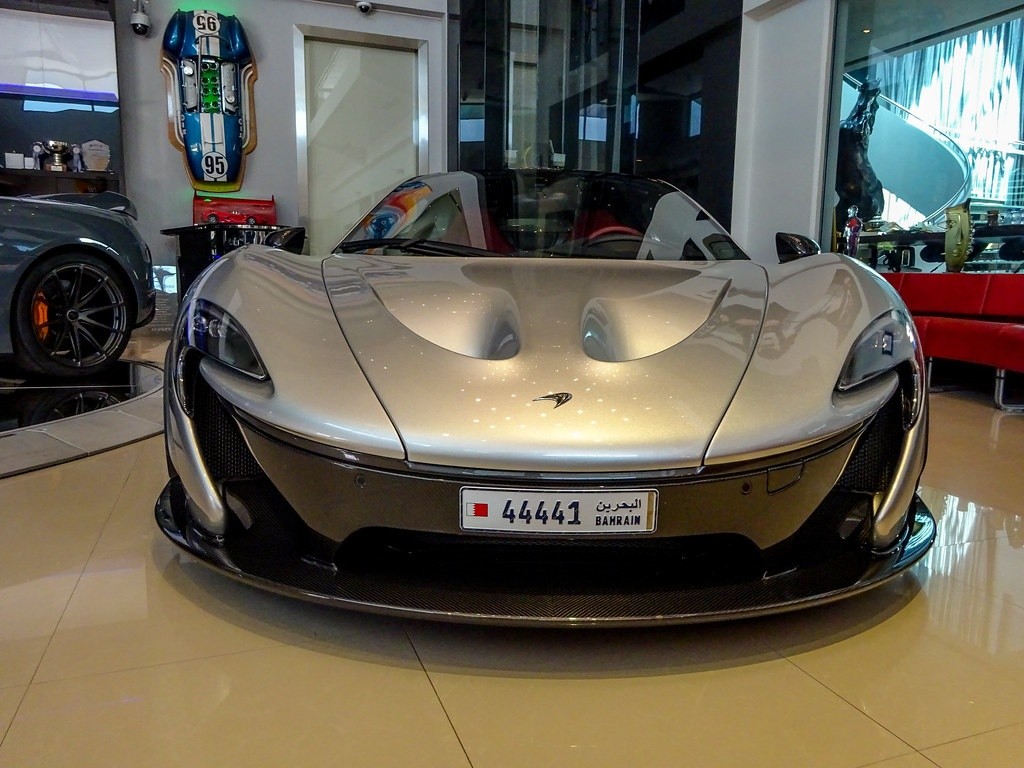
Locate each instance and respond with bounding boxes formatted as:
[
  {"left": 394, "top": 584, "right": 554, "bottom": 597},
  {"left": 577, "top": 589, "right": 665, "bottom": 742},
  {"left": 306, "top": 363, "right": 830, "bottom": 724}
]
[{"left": 872, "top": 264, "right": 1024, "bottom": 416}]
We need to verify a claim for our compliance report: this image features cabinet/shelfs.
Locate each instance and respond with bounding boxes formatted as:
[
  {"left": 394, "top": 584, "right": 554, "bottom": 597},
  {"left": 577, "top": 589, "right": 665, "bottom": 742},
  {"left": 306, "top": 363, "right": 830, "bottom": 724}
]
[
  {"left": 161, "top": 225, "right": 307, "bottom": 308},
  {"left": 0, "top": 166, "right": 118, "bottom": 196}
]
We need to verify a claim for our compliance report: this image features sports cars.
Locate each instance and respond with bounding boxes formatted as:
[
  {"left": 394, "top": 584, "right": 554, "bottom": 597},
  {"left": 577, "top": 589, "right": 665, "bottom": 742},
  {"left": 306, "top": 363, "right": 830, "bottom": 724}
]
[
  {"left": 0, "top": 185, "right": 159, "bottom": 381},
  {"left": 0, "top": 363, "right": 142, "bottom": 437},
  {"left": 852, "top": 218, "right": 906, "bottom": 263},
  {"left": 153, "top": 167, "right": 941, "bottom": 633}
]
